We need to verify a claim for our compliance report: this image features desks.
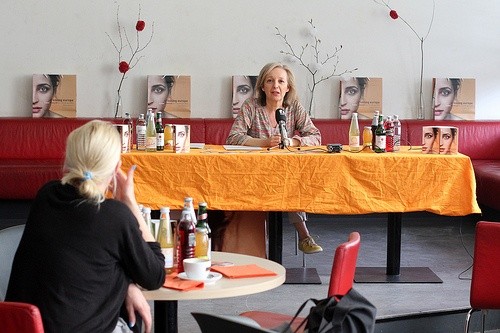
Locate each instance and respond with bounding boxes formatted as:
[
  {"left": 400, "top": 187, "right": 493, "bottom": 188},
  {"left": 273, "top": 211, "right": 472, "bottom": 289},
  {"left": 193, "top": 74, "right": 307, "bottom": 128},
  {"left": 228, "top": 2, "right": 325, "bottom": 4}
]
[
  {"left": 135, "top": 250, "right": 285, "bottom": 333},
  {"left": 106, "top": 146, "right": 482, "bottom": 285}
]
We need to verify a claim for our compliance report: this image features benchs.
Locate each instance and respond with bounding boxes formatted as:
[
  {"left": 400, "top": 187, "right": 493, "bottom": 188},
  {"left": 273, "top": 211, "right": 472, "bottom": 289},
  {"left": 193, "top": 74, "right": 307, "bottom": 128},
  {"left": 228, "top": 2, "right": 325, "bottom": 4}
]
[{"left": 0, "top": 117, "right": 500, "bottom": 201}]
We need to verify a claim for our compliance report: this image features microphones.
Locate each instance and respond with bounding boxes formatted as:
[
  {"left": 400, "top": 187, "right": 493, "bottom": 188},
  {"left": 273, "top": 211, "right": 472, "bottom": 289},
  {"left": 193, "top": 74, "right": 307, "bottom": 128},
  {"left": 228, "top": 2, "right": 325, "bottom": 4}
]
[{"left": 275, "top": 108, "right": 289, "bottom": 146}]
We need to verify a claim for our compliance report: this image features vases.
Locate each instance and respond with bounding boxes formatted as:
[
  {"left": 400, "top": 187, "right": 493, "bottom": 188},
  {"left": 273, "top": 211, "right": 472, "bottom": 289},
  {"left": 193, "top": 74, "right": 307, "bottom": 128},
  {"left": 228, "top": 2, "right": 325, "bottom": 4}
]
[
  {"left": 114, "top": 95, "right": 123, "bottom": 118},
  {"left": 417, "top": 93, "right": 424, "bottom": 119},
  {"left": 306, "top": 94, "right": 316, "bottom": 119}
]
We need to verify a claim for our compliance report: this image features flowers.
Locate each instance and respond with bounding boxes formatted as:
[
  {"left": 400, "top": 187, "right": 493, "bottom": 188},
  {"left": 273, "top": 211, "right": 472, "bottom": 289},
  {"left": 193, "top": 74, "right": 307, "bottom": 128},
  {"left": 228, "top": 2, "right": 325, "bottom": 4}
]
[
  {"left": 374, "top": 0, "right": 435, "bottom": 119},
  {"left": 104, "top": 2, "right": 156, "bottom": 117}
]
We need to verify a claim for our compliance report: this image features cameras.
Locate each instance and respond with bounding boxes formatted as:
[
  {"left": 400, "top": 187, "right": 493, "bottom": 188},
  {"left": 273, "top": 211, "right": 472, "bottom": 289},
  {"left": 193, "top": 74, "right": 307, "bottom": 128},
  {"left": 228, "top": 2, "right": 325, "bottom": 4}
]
[{"left": 327, "top": 142, "right": 342, "bottom": 152}]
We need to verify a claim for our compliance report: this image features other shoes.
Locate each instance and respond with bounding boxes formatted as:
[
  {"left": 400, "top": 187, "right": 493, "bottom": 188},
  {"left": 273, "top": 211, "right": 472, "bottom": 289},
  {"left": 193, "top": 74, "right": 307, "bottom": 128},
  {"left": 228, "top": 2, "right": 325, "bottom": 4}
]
[{"left": 298, "top": 237, "right": 323, "bottom": 254}]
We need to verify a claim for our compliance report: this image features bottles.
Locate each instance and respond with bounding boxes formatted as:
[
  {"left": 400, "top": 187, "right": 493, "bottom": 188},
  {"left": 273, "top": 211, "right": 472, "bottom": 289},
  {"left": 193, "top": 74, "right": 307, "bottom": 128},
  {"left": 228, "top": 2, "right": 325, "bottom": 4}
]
[
  {"left": 371, "top": 116, "right": 379, "bottom": 150},
  {"left": 140, "top": 197, "right": 212, "bottom": 275},
  {"left": 384, "top": 116, "right": 394, "bottom": 152},
  {"left": 375, "top": 115, "right": 387, "bottom": 153},
  {"left": 154, "top": 111, "right": 165, "bottom": 151},
  {"left": 145, "top": 113, "right": 158, "bottom": 152},
  {"left": 392, "top": 114, "right": 402, "bottom": 151},
  {"left": 123, "top": 112, "right": 133, "bottom": 150},
  {"left": 145, "top": 109, "right": 153, "bottom": 127},
  {"left": 376, "top": 111, "right": 379, "bottom": 116},
  {"left": 163, "top": 124, "right": 174, "bottom": 153},
  {"left": 348, "top": 113, "right": 360, "bottom": 151},
  {"left": 363, "top": 126, "right": 372, "bottom": 151},
  {"left": 136, "top": 114, "right": 147, "bottom": 151}
]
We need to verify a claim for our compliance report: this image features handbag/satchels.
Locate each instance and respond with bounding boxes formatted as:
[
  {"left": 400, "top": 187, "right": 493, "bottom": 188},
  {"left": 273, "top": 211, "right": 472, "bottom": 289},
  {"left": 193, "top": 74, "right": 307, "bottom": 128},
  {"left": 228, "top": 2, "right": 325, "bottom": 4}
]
[{"left": 283, "top": 288, "right": 377, "bottom": 333}]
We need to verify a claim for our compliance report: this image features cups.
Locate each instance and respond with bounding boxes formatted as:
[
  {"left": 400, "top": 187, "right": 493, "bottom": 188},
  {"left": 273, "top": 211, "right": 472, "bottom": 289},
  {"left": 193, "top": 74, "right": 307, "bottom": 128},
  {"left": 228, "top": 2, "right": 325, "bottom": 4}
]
[{"left": 182, "top": 257, "right": 211, "bottom": 279}]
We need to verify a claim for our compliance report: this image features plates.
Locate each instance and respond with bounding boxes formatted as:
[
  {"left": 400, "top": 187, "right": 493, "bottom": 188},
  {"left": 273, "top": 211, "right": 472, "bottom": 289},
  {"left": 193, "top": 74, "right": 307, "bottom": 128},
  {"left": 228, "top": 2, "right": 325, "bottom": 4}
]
[{"left": 178, "top": 272, "right": 223, "bottom": 285}]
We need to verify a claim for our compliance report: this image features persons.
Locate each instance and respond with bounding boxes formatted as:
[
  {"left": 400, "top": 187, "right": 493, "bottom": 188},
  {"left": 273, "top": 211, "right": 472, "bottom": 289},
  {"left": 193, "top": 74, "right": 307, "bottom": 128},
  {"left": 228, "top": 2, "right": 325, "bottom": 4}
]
[
  {"left": 32, "top": 74, "right": 64, "bottom": 118},
  {"left": 439, "top": 128, "right": 457, "bottom": 155},
  {"left": 232, "top": 75, "right": 258, "bottom": 119},
  {"left": 422, "top": 127, "right": 440, "bottom": 154},
  {"left": 176, "top": 125, "right": 189, "bottom": 153},
  {"left": 432, "top": 78, "right": 466, "bottom": 121},
  {"left": 147, "top": 75, "right": 178, "bottom": 118},
  {"left": 339, "top": 77, "right": 370, "bottom": 119},
  {"left": 4, "top": 120, "right": 165, "bottom": 333},
  {"left": 122, "top": 126, "right": 129, "bottom": 153},
  {"left": 226, "top": 62, "right": 324, "bottom": 253}
]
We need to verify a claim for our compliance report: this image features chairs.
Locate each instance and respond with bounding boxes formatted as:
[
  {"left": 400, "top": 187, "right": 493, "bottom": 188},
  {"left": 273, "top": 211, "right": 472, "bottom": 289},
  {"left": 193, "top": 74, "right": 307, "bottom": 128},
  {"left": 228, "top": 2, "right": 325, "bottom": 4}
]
[
  {"left": 465, "top": 221, "right": 500, "bottom": 333},
  {"left": 239, "top": 231, "right": 360, "bottom": 333}
]
